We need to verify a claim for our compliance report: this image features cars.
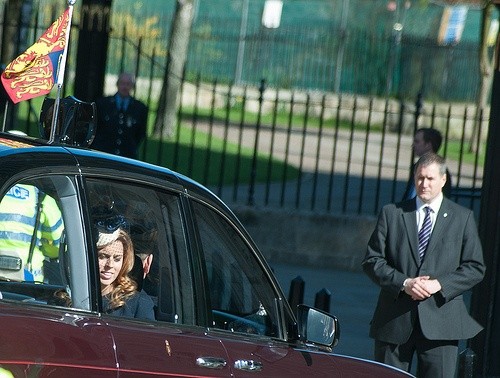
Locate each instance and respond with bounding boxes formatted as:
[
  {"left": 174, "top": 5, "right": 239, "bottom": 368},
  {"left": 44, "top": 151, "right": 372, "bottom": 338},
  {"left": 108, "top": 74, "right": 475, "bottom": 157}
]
[{"left": 0, "top": 141, "right": 416, "bottom": 378}]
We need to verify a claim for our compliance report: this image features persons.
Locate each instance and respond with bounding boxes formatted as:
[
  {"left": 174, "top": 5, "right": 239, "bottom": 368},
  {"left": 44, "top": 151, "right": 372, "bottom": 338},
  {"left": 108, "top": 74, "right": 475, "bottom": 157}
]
[
  {"left": 402, "top": 127, "right": 452, "bottom": 202},
  {"left": 93, "top": 205, "right": 156, "bottom": 320},
  {"left": 97, "top": 72, "right": 149, "bottom": 160},
  {"left": 363, "top": 152, "right": 486, "bottom": 378}
]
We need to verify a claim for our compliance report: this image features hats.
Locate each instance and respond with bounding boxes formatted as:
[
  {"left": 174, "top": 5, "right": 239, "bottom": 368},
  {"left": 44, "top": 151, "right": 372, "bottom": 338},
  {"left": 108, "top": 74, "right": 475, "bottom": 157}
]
[{"left": 94, "top": 211, "right": 125, "bottom": 249}]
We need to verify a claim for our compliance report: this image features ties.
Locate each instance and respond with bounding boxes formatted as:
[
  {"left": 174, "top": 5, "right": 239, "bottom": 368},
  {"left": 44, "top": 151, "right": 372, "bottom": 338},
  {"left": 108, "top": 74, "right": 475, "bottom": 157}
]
[
  {"left": 408, "top": 179, "right": 414, "bottom": 200},
  {"left": 121, "top": 99, "right": 125, "bottom": 112},
  {"left": 418, "top": 206, "right": 435, "bottom": 264}
]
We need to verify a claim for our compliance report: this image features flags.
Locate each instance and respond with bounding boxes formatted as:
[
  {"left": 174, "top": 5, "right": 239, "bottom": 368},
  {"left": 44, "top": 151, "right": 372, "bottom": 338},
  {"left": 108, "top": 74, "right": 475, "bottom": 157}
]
[{"left": 1, "top": 6, "right": 71, "bottom": 104}]
